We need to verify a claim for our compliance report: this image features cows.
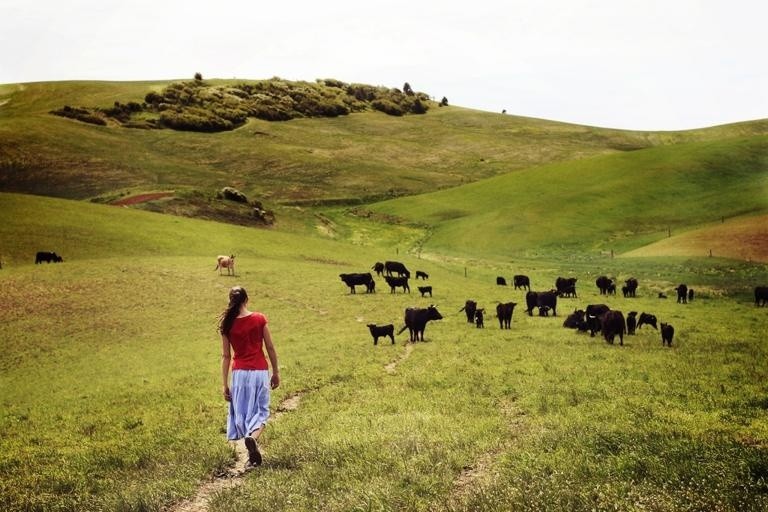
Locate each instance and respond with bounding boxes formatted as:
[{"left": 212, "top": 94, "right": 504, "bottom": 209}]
[
  {"left": 754, "top": 286, "right": 768, "bottom": 307},
  {"left": 398, "top": 304, "right": 443, "bottom": 344},
  {"left": 595, "top": 275, "right": 637, "bottom": 298},
  {"left": 338, "top": 261, "right": 435, "bottom": 298},
  {"left": 497, "top": 276, "right": 506, "bottom": 285},
  {"left": 658, "top": 292, "right": 667, "bottom": 299},
  {"left": 674, "top": 285, "right": 694, "bottom": 304},
  {"left": 660, "top": 322, "right": 675, "bottom": 347},
  {"left": 458, "top": 300, "right": 485, "bottom": 328},
  {"left": 562, "top": 304, "right": 658, "bottom": 349},
  {"left": 496, "top": 302, "right": 517, "bottom": 330},
  {"left": 214, "top": 254, "right": 236, "bottom": 276},
  {"left": 513, "top": 274, "right": 578, "bottom": 317},
  {"left": 367, "top": 322, "right": 395, "bottom": 346},
  {"left": 35, "top": 251, "right": 64, "bottom": 264}
]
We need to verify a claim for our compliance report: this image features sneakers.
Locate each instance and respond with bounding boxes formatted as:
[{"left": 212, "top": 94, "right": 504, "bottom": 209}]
[{"left": 244, "top": 435, "right": 263, "bottom": 467}]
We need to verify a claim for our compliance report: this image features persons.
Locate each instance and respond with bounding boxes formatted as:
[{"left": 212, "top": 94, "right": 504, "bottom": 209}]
[{"left": 215, "top": 285, "right": 280, "bottom": 467}]
